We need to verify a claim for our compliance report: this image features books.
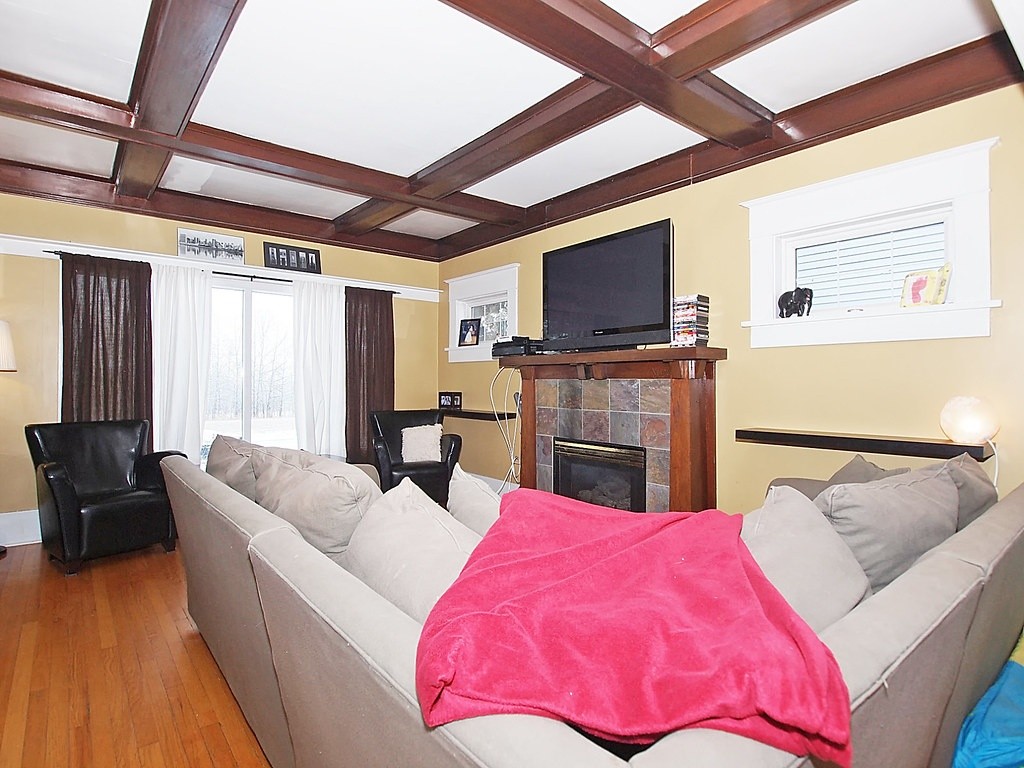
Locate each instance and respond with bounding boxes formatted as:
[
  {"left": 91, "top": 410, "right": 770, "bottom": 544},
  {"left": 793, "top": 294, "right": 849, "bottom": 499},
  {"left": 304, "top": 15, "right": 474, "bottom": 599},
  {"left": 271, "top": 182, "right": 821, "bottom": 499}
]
[{"left": 670, "top": 294, "right": 709, "bottom": 347}]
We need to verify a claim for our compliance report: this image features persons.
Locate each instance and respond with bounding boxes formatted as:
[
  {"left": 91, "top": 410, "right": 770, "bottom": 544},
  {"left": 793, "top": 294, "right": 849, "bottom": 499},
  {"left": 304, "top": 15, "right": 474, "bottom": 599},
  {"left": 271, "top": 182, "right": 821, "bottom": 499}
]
[{"left": 463, "top": 322, "right": 474, "bottom": 342}]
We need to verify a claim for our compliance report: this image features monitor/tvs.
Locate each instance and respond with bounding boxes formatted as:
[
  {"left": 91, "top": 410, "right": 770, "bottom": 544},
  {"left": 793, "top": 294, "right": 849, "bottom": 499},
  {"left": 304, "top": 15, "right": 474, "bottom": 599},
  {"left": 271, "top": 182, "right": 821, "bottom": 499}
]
[{"left": 542, "top": 217, "right": 675, "bottom": 353}]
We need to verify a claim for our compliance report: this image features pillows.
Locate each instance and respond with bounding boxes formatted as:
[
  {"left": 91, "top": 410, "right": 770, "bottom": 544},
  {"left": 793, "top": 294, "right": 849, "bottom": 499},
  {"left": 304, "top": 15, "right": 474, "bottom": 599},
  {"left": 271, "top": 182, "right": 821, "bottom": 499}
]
[
  {"left": 251, "top": 447, "right": 384, "bottom": 561},
  {"left": 401, "top": 424, "right": 443, "bottom": 462},
  {"left": 348, "top": 477, "right": 484, "bottom": 627},
  {"left": 812, "top": 452, "right": 998, "bottom": 596},
  {"left": 206, "top": 433, "right": 264, "bottom": 504},
  {"left": 820, "top": 454, "right": 911, "bottom": 492},
  {"left": 446, "top": 463, "right": 873, "bottom": 634}
]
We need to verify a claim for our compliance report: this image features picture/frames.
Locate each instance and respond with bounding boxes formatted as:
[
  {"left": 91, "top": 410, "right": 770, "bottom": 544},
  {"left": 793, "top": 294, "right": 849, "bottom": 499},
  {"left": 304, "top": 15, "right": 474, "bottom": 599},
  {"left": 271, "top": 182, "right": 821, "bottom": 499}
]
[{"left": 458, "top": 318, "right": 481, "bottom": 347}]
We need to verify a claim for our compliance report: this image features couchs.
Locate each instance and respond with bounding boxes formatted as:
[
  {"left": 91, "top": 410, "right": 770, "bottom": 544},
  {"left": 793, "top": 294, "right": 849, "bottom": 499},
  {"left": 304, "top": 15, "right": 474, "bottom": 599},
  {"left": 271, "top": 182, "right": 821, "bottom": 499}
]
[
  {"left": 766, "top": 479, "right": 1024, "bottom": 768},
  {"left": 160, "top": 456, "right": 380, "bottom": 768},
  {"left": 25, "top": 419, "right": 188, "bottom": 577},
  {"left": 369, "top": 409, "right": 462, "bottom": 512},
  {"left": 249, "top": 529, "right": 988, "bottom": 768}
]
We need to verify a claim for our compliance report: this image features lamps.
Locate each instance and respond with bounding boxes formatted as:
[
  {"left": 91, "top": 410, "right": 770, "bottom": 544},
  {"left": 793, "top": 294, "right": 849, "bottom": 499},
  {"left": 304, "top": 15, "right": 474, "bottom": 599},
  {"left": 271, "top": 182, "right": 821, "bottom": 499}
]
[{"left": 0, "top": 320, "right": 17, "bottom": 558}]
